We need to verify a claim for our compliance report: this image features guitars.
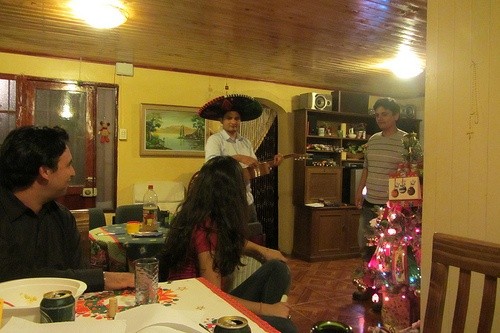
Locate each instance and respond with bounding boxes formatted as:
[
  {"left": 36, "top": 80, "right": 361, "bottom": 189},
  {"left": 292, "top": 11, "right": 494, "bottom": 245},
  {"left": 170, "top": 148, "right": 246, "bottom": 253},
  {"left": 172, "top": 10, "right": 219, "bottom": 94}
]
[{"left": 244, "top": 152, "right": 312, "bottom": 180}]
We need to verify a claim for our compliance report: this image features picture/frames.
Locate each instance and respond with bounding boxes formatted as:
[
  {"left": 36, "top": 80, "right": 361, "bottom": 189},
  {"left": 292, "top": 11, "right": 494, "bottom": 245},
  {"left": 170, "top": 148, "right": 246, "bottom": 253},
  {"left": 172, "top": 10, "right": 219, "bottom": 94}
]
[{"left": 140, "top": 102, "right": 210, "bottom": 158}]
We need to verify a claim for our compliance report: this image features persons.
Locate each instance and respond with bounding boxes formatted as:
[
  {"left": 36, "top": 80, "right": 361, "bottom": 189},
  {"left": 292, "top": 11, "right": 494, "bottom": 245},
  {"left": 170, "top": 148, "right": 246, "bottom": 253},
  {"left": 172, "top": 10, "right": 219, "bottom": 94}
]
[
  {"left": 165, "top": 155, "right": 299, "bottom": 333},
  {"left": 198, "top": 95, "right": 282, "bottom": 247},
  {"left": 353, "top": 96, "right": 417, "bottom": 311},
  {"left": 0, "top": 125, "right": 151, "bottom": 293}
]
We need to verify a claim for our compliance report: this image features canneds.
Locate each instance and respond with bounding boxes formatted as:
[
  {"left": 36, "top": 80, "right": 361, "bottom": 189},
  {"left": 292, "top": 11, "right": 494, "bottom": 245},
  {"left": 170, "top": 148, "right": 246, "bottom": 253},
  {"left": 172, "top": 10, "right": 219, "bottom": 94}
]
[
  {"left": 40, "top": 290, "right": 75, "bottom": 323},
  {"left": 213, "top": 316, "right": 252, "bottom": 333}
]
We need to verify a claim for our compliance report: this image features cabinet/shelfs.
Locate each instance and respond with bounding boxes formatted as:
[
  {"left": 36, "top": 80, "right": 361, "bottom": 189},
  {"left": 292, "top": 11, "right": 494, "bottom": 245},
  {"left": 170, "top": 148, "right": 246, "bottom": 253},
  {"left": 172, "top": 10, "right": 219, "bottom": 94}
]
[
  {"left": 294, "top": 108, "right": 376, "bottom": 208},
  {"left": 294, "top": 206, "right": 363, "bottom": 262}
]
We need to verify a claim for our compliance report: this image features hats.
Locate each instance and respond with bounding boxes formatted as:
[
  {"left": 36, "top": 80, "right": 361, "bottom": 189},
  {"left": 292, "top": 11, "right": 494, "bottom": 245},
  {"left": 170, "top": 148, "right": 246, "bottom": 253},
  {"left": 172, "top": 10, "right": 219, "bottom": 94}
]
[{"left": 198, "top": 94, "right": 261, "bottom": 121}]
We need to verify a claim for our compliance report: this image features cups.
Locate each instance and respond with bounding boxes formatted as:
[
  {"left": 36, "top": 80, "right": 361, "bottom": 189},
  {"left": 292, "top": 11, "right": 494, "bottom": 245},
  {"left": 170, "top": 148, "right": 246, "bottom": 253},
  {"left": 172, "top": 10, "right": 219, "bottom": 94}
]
[
  {"left": 133, "top": 257, "right": 159, "bottom": 306},
  {"left": 126, "top": 222, "right": 142, "bottom": 235},
  {"left": 318, "top": 128, "right": 325, "bottom": 136}
]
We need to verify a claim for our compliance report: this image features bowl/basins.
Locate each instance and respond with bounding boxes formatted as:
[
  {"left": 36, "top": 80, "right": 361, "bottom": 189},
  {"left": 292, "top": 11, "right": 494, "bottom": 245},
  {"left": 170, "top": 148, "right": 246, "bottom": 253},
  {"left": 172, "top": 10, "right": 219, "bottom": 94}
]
[
  {"left": 348, "top": 134, "right": 356, "bottom": 138},
  {"left": 0, "top": 277, "right": 87, "bottom": 322}
]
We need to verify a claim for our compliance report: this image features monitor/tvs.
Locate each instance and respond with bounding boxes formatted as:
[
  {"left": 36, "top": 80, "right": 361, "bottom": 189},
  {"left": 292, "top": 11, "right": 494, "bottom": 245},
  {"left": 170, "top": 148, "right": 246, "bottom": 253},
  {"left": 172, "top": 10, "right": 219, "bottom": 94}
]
[{"left": 342, "top": 167, "right": 364, "bottom": 205}]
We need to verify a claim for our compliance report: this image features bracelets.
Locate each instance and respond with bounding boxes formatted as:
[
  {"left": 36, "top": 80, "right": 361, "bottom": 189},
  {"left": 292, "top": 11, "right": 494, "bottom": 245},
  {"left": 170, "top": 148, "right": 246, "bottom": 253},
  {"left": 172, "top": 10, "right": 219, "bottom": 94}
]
[{"left": 260, "top": 302, "right": 262, "bottom": 317}]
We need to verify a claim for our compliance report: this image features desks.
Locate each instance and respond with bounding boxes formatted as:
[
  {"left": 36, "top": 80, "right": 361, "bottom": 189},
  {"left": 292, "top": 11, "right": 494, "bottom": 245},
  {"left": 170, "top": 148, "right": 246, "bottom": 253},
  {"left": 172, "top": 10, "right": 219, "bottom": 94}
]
[
  {"left": 75, "top": 277, "right": 282, "bottom": 333},
  {"left": 88, "top": 221, "right": 170, "bottom": 282}
]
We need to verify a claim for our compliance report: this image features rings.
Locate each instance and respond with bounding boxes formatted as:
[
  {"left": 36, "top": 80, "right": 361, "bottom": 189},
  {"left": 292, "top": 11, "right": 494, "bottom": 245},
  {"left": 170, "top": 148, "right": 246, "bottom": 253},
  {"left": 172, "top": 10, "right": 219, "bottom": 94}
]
[{"left": 289, "top": 315, "right": 291, "bottom": 319}]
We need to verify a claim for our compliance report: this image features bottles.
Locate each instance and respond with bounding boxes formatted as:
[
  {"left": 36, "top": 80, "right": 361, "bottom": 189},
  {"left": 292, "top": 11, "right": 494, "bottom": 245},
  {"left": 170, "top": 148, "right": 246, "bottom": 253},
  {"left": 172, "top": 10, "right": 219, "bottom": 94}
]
[{"left": 142, "top": 185, "right": 158, "bottom": 232}]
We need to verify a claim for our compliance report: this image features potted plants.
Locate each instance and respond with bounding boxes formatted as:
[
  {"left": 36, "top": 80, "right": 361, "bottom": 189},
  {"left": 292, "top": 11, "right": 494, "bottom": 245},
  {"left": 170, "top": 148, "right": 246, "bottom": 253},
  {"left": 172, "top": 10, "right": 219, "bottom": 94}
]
[{"left": 344, "top": 142, "right": 367, "bottom": 161}]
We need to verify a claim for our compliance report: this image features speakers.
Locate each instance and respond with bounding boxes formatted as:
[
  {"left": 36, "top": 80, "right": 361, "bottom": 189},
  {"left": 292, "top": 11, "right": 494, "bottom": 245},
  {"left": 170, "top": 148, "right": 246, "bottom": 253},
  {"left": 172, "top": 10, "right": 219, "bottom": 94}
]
[
  {"left": 331, "top": 90, "right": 369, "bottom": 114},
  {"left": 299, "top": 92, "right": 333, "bottom": 111}
]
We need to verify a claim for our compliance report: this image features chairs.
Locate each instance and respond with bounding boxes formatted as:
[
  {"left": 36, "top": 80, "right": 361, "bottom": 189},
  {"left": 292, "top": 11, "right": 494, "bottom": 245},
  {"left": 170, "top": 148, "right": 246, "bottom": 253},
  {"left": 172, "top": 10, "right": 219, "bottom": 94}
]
[
  {"left": 89, "top": 207, "right": 106, "bottom": 230},
  {"left": 423, "top": 233, "right": 500, "bottom": 333},
  {"left": 112, "top": 204, "right": 161, "bottom": 225}
]
